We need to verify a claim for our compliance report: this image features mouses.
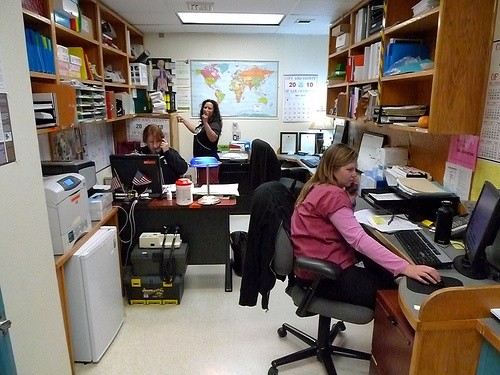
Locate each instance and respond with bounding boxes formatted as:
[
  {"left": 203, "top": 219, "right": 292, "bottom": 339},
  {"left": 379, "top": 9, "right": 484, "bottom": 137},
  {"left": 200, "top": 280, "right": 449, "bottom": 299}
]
[{"left": 423, "top": 275, "right": 444, "bottom": 286}]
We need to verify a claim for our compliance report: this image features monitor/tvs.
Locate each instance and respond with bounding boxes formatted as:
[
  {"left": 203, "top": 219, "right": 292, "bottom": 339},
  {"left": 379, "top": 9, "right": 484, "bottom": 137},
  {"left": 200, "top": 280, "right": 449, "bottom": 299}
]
[
  {"left": 354, "top": 130, "right": 387, "bottom": 175},
  {"left": 331, "top": 124, "right": 345, "bottom": 145},
  {"left": 109, "top": 154, "right": 163, "bottom": 206},
  {"left": 453, "top": 180, "right": 500, "bottom": 278}
]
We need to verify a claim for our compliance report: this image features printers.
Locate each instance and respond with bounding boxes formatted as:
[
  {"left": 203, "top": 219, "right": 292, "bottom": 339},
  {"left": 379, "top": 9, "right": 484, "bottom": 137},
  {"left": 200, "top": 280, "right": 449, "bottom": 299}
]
[{"left": 39, "top": 159, "right": 112, "bottom": 220}]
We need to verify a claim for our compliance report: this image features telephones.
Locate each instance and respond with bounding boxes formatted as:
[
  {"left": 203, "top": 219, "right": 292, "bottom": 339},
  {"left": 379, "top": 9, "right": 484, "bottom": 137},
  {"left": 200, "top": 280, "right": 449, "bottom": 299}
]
[
  {"left": 156, "top": 135, "right": 165, "bottom": 153},
  {"left": 347, "top": 168, "right": 358, "bottom": 194}
]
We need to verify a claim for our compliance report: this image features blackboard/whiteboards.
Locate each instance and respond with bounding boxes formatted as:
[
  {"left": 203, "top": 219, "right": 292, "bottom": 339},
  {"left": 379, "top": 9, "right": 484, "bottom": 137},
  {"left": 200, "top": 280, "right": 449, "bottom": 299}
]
[{"left": 133, "top": 57, "right": 172, "bottom": 113}]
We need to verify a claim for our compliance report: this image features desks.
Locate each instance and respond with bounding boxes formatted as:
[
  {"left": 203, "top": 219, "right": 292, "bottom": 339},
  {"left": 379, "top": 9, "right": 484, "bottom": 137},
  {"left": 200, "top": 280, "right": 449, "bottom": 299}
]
[
  {"left": 53, "top": 203, "right": 126, "bottom": 375},
  {"left": 217, "top": 148, "right": 500, "bottom": 375},
  {"left": 112, "top": 184, "right": 238, "bottom": 293}
]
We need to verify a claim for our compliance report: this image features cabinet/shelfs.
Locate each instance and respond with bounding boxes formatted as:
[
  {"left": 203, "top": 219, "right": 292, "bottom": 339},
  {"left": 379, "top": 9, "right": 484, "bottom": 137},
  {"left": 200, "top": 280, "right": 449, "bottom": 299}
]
[
  {"left": 21, "top": 0, "right": 180, "bottom": 155},
  {"left": 326, "top": 0, "right": 498, "bottom": 136},
  {"left": 367, "top": 289, "right": 416, "bottom": 375}
]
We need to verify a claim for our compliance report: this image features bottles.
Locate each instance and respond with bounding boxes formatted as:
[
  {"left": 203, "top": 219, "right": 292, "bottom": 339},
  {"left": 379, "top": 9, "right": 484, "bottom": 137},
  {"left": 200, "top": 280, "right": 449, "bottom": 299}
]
[
  {"left": 434, "top": 201, "right": 454, "bottom": 246},
  {"left": 176, "top": 178, "right": 195, "bottom": 206}
]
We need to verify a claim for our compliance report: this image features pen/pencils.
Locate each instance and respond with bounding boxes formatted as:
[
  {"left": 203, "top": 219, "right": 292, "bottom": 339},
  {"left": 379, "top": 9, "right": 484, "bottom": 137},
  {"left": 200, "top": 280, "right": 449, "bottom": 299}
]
[{"left": 388, "top": 218, "right": 394, "bottom": 225}]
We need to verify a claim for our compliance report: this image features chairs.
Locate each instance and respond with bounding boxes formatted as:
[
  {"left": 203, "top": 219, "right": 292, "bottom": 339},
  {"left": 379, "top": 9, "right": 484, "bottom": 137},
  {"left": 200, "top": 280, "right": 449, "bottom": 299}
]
[
  {"left": 244, "top": 139, "right": 310, "bottom": 214},
  {"left": 238, "top": 180, "right": 376, "bottom": 375}
]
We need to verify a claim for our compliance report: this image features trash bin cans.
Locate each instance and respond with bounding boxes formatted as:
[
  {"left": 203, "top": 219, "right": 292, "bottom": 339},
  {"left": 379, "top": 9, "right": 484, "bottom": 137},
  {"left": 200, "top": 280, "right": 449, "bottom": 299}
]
[{"left": 230, "top": 231, "right": 248, "bottom": 276}]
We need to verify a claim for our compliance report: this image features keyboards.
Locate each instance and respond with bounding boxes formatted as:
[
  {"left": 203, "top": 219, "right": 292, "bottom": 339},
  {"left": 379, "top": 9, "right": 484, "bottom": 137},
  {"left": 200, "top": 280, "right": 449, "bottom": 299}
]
[
  {"left": 303, "top": 158, "right": 319, "bottom": 167},
  {"left": 394, "top": 229, "right": 453, "bottom": 269}
]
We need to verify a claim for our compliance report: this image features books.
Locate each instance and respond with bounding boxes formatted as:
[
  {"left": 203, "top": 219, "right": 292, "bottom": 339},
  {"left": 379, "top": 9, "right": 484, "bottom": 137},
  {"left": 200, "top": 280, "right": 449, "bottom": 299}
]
[{"left": 388, "top": 116, "right": 419, "bottom": 126}]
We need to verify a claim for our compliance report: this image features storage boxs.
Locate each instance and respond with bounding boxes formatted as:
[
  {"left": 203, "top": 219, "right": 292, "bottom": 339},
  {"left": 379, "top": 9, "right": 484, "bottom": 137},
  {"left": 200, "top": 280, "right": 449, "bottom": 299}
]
[
  {"left": 69, "top": 54, "right": 82, "bottom": 67},
  {"left": 59, "top": 69, "right": 69, "bottom": 77},
  {"left": 53, "top": 10, "right": 71, "bottom": 29},
  {"left": 68, "top": 70, "right": 81, "bottom": 78},
  {"left": 57, "top": 60, "right": 69, "bottom": 70},
  {"left": 332, "top": 24, "right": 350, "bottom": 37},
  {"left": 56, "top": 43, "right": 69, "bottom": 55},
  {"left": 57, "top": 52, "right": 70, "bottom": 63},
  {"left": 68, "top": 63, "right": 81, "bottom": 72},
  {"left": 336, "top": 32, "right": 350, "bottom": 51},
  {"left": 53, "top": 0, "right": 79, "bottom": 19}
]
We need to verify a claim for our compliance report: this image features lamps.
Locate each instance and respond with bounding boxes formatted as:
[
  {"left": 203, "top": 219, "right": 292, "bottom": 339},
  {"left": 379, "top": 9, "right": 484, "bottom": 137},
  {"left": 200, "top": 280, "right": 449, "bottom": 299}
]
[{"left": 187, "top": 156, "right": 223, "bottom": 205}]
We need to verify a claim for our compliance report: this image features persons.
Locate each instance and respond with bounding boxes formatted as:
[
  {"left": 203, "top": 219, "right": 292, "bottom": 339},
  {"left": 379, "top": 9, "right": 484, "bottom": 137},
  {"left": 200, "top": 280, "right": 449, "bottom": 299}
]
[
  {"left": 176, "top": 99, "right": 222, "bottom": 184},
  {"left": 139, "top": 124, "right": 188, "bottom": 186},
  {"left": 294, "top": 143, "right": 440, "bottom": 309}
]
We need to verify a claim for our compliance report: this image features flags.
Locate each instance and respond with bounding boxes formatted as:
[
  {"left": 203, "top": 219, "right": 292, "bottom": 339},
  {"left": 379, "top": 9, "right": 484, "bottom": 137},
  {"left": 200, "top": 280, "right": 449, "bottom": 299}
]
[
  {"left": 111, "top": 170, "right": 121, "bottom": 190},
  {"left": 132, "top": 171, "right": 151, "bottom": 185}
]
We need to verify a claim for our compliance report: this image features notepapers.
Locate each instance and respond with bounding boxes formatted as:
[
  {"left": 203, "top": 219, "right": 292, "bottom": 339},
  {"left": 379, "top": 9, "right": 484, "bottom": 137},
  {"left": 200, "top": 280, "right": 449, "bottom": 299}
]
[
  {"left": 422, "top": 220, "right": 433, "bottom": 227},
  {"left": 373, "top": 217, "right": 385, "bottom": 224}
]
